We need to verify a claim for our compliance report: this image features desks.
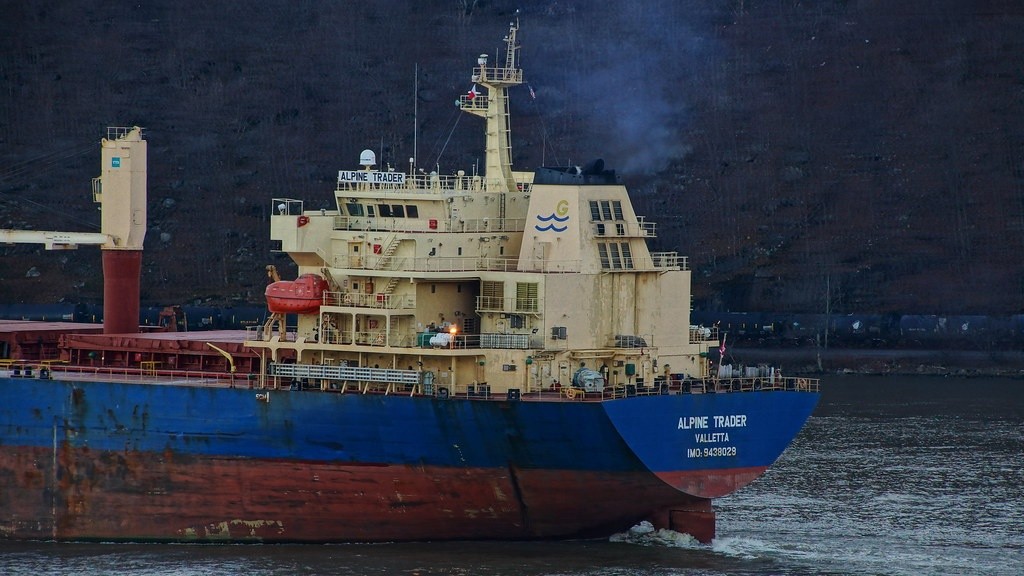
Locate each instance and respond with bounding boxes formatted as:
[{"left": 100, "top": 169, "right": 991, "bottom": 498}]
[
  {"left": 0, "top": 358, "right": 71, "bottom": 371},
  {"left": 140, "top": 361, "right": 166, "bottom": 376}
]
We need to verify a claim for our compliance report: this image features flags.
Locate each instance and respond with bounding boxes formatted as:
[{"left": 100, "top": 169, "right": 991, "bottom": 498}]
[
  {"left": 468, "top": 85, "right": 476, "bottom": 99},
  {"left": 528, "top": 85, "right": 535, "bottom": 97},
  {"left": 719, "top": 339, "right": 726, "bottom": 356}
]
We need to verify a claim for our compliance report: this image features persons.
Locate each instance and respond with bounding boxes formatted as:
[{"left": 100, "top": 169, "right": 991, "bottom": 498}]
[{"left": 776, "top": 369, "right": 781, "bottom": 380}]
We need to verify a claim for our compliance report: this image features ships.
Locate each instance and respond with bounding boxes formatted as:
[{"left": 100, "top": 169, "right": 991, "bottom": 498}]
[{"left": 0, "top": 9, "right": 820, "bottom": 543}]
